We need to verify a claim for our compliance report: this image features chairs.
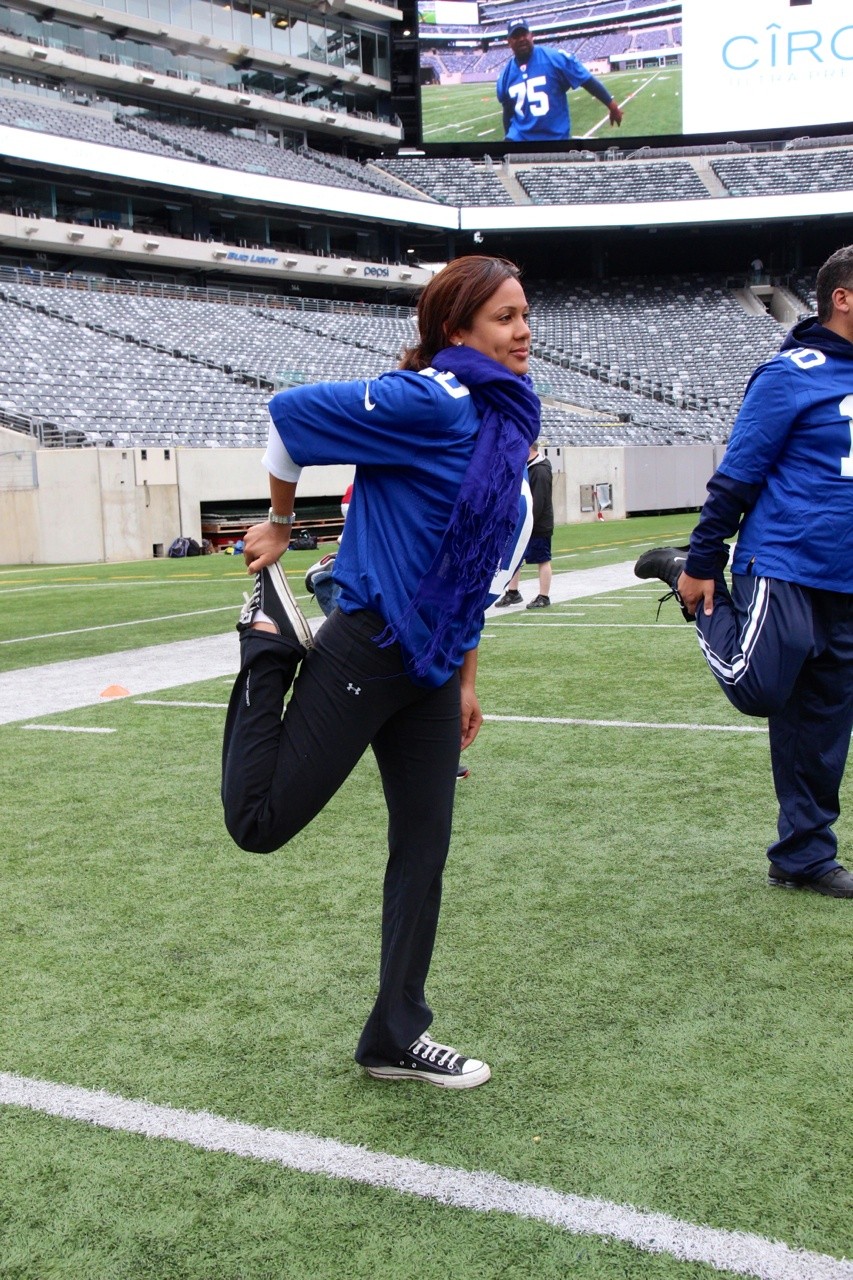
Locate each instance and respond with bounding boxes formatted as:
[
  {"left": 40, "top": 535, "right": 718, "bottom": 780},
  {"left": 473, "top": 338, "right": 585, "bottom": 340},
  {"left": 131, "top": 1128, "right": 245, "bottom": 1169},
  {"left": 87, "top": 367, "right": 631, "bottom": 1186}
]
[
  {"left": 1, "top": 266, "right": 819, "bottom": 449},
  {"left": 1, "top": 97, "right": 853, "bottom": 208}
]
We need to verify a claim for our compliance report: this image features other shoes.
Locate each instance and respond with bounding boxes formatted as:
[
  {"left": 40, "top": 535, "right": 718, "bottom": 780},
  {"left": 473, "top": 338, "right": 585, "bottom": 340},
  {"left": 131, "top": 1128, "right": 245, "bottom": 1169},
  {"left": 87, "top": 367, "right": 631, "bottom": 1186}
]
[
  {"left": 456, "top": 765, "right": 468, "bottom": 778},
  {"left": 305, "top": 553, "right": 339, "bottom": 593}
]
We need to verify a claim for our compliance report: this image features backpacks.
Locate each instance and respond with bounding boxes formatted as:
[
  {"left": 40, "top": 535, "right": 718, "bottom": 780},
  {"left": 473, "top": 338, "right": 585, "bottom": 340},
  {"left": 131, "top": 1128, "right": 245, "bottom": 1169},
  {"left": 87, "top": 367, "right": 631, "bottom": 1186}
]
[
  {"left": 169, "top": 537, "right": 190, "bottom": 557},
  {"left": 188, "top": 540, "right": 201, "bottom": 556}
]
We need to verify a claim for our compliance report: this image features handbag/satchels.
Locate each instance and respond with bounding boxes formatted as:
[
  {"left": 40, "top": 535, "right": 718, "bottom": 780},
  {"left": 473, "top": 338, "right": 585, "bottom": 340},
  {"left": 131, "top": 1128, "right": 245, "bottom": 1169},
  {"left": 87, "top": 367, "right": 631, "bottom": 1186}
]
[
  {"left": 240, "top": 559, "right": 315, "bottom": 652},
  {"left": 291, "top": 530, "right": 315, "bottom": 550}
]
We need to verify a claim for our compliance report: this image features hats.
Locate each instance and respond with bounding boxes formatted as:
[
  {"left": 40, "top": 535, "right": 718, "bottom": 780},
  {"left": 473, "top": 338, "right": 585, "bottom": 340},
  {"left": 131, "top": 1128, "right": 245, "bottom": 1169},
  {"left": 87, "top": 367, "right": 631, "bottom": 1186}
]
[{"left": 508, "top": 18, "right": 529, "bottom": 36}]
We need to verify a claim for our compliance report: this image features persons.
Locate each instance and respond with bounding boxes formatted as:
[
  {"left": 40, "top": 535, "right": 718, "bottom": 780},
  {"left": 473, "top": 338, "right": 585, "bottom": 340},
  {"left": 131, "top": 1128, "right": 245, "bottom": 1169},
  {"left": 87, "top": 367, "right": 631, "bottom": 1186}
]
[
  {"left": 497, "top": 19, "right": 623, "bottom": 145},
  {"left": 221, "top": 255, "right": 535, "bottom": 1089},
  {"left": 496, "top": 441, "right": 554, "bottom": 609},
  {"left": 635, "top": 244, "right": 853, "bottom": 901}
]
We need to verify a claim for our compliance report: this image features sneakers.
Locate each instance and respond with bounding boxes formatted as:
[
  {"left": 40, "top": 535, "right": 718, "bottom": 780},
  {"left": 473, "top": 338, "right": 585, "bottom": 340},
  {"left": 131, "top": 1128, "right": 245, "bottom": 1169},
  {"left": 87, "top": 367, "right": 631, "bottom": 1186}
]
[
  {"left": 635, "top": 543, "right": 730, "bottom": 620},
  {"left": 769, "top": 861, "right": 853, "bottom": 898},
  {"left": 494, "top": 591, "right": 523, "bottom": 607},
  {"left": 527, "top": 595, "right": 550, "bottom": 609},
  {"left": 368, "top": 1030, "right": 491, "bottom": 1088}
]
